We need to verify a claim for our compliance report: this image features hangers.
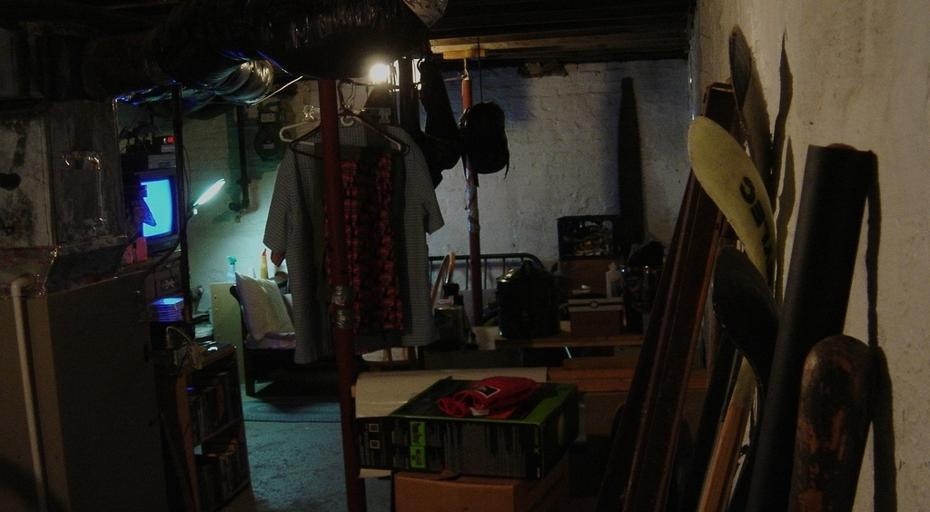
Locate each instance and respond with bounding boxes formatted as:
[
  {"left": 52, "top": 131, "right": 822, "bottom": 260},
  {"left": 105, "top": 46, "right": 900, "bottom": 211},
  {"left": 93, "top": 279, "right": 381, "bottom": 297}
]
[{"left": 277, "top": 80, "right": 415, "bottom": 161}]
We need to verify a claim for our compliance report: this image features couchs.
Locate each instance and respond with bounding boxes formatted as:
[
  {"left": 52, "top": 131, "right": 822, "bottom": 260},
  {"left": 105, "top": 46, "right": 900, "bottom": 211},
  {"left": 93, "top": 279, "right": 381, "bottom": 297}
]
[{"left": 229, "top": 252, "right": 546, "bottom": 395}]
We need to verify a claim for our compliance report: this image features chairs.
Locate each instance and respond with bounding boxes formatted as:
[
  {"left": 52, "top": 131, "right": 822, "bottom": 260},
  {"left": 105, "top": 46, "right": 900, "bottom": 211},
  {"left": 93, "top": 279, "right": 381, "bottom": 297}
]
[{"left": 361, "top": 249, "right": 458, "bottom": 372}]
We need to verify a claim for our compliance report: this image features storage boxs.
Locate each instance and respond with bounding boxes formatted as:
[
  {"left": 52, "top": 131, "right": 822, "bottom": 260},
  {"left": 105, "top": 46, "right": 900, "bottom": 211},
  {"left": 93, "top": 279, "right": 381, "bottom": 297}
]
[
  {"left": 355, "top": 377, "right": 580, "bottom": 480},
  {"left": 393, "top": 450, "right": 573, "bottom": 512}
]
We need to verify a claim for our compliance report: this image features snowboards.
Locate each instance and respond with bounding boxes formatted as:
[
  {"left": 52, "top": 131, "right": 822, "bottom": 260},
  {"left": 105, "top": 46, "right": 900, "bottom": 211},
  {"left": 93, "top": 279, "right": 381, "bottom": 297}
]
[{"left": 686, "top": 23, "right": 777, "bottom": 511}]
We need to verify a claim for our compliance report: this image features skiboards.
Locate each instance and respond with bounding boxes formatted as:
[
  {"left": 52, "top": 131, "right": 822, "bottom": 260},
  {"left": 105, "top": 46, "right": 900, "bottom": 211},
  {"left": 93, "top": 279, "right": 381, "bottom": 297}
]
[{"left": 589, "top": 82, "right": 736, "bottom": 511}]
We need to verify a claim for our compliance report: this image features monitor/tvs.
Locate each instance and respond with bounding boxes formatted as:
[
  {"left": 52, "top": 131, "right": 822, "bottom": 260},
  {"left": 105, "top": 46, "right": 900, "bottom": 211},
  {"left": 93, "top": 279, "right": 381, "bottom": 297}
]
[{"left": 136, "top": 166, "right": 178, "bottom": 253}]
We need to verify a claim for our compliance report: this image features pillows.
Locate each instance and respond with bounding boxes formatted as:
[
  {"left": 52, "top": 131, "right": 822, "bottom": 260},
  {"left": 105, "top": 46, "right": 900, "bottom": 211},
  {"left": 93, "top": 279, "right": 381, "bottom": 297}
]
[{"left": 236, "top": 273, "right": 297, "bottom": 342}]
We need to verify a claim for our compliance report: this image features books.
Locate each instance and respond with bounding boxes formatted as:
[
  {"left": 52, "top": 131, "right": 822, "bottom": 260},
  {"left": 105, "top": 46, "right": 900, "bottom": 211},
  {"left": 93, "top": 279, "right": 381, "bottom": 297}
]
[{"left": 185, "top": 364, "right": 243, "bottom": 505}]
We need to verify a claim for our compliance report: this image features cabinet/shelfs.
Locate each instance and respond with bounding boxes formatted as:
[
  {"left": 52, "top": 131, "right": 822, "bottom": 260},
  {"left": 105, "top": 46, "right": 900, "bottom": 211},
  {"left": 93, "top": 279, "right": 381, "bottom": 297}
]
[
  {"left": 153, "top": 339, "right": 252, "bottom": 512},
  {"left": 142, "top": 250, "right": 182, "bottom": 316}
]
